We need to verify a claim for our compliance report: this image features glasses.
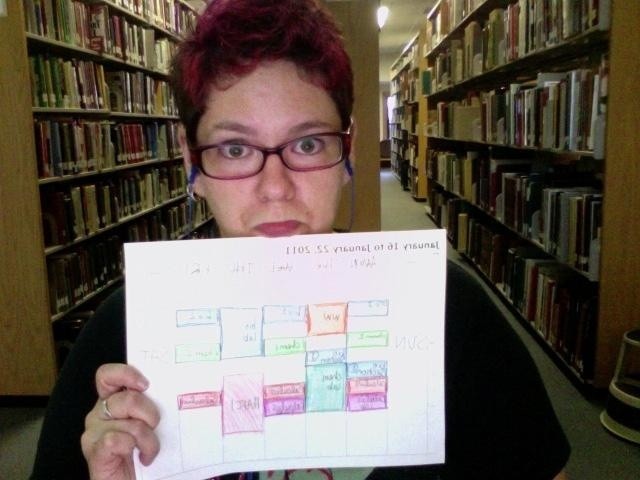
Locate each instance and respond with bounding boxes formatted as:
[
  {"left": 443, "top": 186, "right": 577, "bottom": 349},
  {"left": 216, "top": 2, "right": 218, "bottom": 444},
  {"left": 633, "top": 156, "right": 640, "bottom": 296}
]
[{"left": 187, "top": 124, "right": 352, "bottom": 181}]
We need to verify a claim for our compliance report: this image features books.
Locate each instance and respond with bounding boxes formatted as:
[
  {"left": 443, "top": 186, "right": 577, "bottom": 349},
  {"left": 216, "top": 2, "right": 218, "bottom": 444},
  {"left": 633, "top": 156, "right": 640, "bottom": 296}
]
[
  {"left": 386, "top": 0, "right": 613, "bottom": 380},
  {"left": 9, "top": 1, "right": 220, "bottom": 365}
]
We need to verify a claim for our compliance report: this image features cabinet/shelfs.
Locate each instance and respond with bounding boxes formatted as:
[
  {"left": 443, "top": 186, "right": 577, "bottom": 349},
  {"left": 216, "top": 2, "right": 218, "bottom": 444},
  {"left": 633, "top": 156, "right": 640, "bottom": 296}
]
[
  {"left": 1, "top": 0, "right": 217, "bottom": 395},
  {"left": 389, "top": 2, "right": 639, "bottom": 409}
]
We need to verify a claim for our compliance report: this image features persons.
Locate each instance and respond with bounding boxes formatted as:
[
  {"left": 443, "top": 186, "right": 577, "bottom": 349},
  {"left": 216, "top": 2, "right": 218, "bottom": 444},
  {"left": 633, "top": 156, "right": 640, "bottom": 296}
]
[{"left": 28, "top": 3, "right": 578, "bottom": 479}]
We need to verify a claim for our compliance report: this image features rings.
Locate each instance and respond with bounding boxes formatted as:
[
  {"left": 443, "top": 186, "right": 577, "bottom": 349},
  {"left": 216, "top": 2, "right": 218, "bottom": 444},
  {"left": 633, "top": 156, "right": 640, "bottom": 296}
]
[{"left": 100, "top": 400, "right": 116, "bottom": 419}]
